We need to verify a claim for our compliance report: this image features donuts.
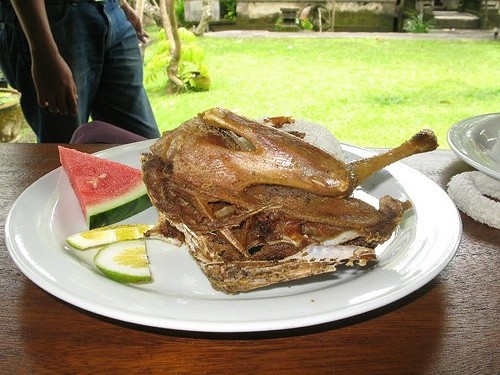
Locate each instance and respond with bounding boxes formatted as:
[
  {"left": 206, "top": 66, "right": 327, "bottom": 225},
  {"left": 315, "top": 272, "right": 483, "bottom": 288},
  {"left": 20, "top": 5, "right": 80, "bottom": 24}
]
[{"left": 447, "top": 170, "right": 500, "bottom": 230}]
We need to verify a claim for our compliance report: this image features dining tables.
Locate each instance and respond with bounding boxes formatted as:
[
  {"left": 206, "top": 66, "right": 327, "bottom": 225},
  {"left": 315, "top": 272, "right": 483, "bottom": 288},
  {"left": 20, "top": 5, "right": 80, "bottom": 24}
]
[{"left": 0, "top": 142, "right": 500, "bottom": 375}]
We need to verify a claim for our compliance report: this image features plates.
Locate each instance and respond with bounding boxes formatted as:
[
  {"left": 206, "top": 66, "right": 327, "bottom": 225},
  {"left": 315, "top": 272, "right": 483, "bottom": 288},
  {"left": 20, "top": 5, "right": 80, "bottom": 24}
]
[
  {"left": 3, "top": 137, "right": 463, "bottom": 334},
  {"left": 447, "top": 112, "right": 500, "bottom": 180}
]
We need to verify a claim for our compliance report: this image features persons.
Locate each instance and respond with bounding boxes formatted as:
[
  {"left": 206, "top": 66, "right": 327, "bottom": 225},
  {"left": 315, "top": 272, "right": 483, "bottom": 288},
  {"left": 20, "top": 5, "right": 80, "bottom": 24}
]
[{"left": 1, "top": 0, "right": 161, "bottom": 144}]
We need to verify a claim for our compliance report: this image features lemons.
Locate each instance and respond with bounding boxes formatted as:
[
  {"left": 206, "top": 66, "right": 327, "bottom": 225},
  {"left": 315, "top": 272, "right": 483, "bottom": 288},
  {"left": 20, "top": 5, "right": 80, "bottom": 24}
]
[{"left": 67, "top": 224, "right": 156, "bottom": 284}]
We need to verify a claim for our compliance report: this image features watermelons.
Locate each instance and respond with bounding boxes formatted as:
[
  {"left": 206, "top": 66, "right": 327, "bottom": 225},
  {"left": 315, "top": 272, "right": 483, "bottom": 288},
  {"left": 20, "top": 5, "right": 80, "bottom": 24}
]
[{"left": 57, "top": 144, "right": 152, "bottom": 228}]
